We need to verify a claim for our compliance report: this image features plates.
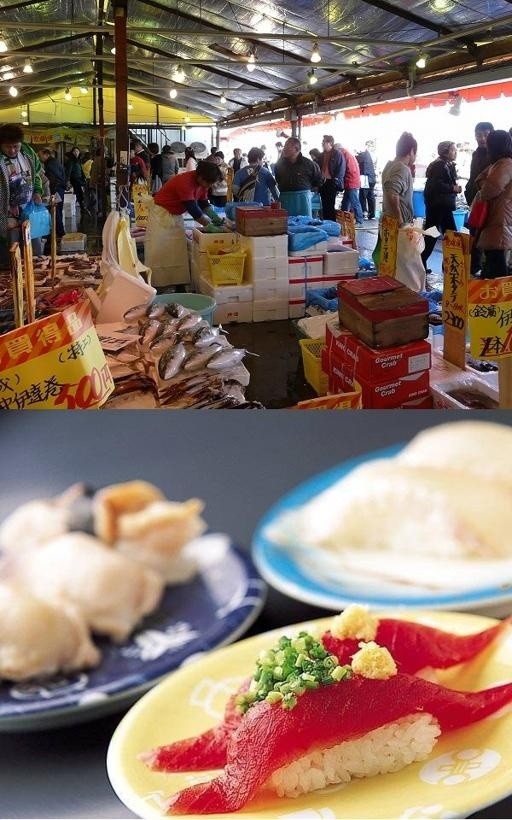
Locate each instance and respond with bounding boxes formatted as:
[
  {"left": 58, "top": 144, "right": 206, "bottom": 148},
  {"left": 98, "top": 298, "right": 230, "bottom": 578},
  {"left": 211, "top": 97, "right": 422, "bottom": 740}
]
[
  {"left": 250, "top": 443, "right": 512, "bottom": 617},
  {"left": 2, "top": 523, "right": 267, "bottom": 733},
  {"left": 103, "top": 620, "right": 511, "bottom": 820}
]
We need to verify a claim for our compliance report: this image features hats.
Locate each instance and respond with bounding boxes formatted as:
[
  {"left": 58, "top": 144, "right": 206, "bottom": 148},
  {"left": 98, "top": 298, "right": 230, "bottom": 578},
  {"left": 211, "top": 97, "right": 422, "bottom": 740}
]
[{"left": 438, "top": 140, "right": 455, "bottom": 157}]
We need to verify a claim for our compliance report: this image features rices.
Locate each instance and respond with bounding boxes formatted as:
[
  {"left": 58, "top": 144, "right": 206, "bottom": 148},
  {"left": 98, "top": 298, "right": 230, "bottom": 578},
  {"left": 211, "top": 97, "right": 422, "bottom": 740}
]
[{"left": 267, "top": 668, "right": 440, "bottom": 799}]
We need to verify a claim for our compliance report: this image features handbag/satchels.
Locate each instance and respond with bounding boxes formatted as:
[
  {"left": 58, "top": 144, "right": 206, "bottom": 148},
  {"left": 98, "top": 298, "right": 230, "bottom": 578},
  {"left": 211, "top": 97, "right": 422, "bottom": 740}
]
[
  {"left": 467, "top": 192, "right": 489, "bottom": 229},
  {"left": 430, "top": 188, "right": 457, "bottom": 211},
  {"left": 236, "top": 165, "right": 262, "bottom": 204}
]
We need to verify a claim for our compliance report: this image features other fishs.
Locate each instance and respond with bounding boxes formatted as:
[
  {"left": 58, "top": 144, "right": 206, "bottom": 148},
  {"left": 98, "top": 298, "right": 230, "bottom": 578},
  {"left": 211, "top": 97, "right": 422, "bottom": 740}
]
[{"left": 122, "top": 301, "right": 261, "bottom": 382}]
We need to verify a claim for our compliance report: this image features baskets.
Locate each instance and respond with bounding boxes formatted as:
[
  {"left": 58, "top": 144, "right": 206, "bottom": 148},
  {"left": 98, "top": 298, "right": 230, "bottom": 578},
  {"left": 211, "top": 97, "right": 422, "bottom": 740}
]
[{"left": 206, "top": 242, "right": 247, "bottom": 288}]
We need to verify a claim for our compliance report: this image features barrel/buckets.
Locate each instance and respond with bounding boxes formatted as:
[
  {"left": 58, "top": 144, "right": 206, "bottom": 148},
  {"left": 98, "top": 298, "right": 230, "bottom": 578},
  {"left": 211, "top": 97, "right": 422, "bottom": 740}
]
[
  {"left": 412, "top": 190, "right": 426, "bottom": 218},
  {"left": 153, "top": 292, "right": 217, "bottom": 328},
  {"left": 452, "top": 209, "right": 468, "bottom": 230}
]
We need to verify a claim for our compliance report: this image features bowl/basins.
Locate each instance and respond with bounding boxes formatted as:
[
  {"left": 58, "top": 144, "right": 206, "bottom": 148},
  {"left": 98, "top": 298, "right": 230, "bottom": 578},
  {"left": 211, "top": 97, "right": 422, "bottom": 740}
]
[{"left": 452, "top": 208, "right": 465, "bottom": 231}]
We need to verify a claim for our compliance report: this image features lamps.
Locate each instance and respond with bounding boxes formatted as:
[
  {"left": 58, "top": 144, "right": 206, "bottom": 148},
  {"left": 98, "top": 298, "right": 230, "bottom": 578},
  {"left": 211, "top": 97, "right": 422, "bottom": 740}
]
[
  {"left": 310, "top": 50, "right": 322, "bottom": 64},
  {"left": 416, "top": 59, "right": 425, "bottom": 70},
  {"left": 246, "top": 51, "right": 256, "bottom": 72},
  {"left": 170, "top": 87, "right": 178, "bottom": 99},
  {"left": 221, "top": 96, "right": 226, "bottom": 104},
  {"left": 0, "top": 40, "right": 115, "bottom": 116},
  {"left": 309, "top": 72, "right": 318, "bottom": 85}
]
